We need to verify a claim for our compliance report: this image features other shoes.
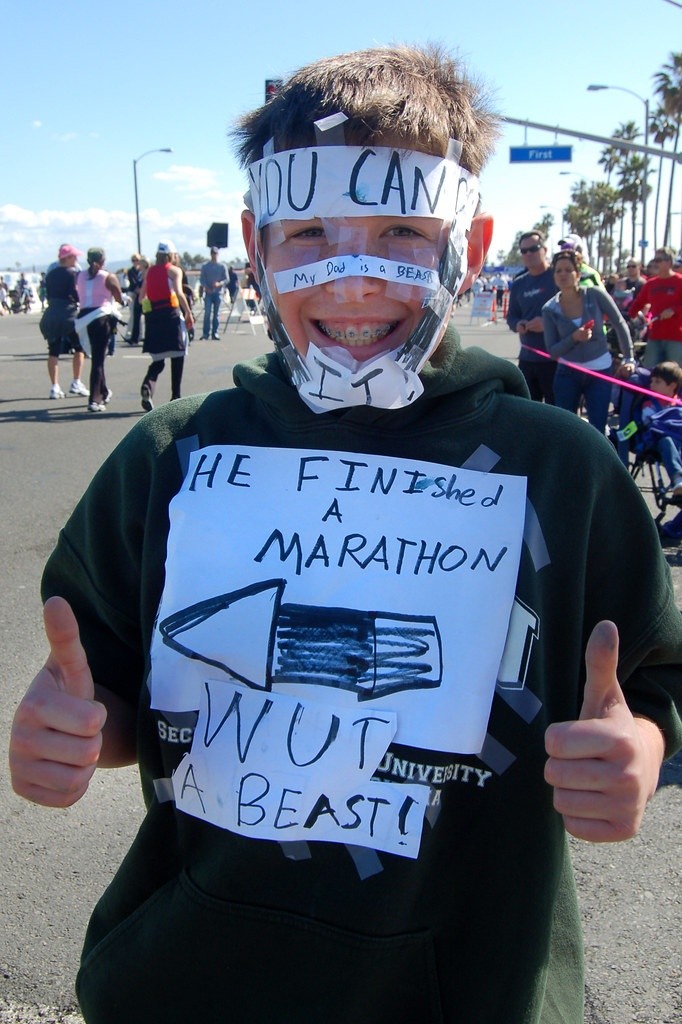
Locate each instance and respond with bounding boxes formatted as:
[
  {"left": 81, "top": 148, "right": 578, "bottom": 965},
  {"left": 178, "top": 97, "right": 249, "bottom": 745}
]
[
  {"left": 200, "top": 335, "right": 209, "bottom": 341},
  {"left": 212, "top": 332, "right": 219, "bottom": 340}
]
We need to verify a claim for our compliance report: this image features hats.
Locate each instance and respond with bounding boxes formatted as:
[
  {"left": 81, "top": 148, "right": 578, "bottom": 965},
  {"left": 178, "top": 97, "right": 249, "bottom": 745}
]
[
  {"left": 157, "top": 243, "right": 170, "bottom": 254},
  {"left": 211, "top": 246, "right": 218, "bottom": 254},
  {"left": 87, "top": 248, "right": 104, "bottom": 263},
  {"left": 558, "top": 234, "right": 582, "bottom": 254},
  {"left": 59, "top": 245, "right": 79, "bottom": 259}
]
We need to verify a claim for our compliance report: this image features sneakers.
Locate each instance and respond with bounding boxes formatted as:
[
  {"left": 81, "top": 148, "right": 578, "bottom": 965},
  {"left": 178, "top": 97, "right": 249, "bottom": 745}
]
[
  {"left": 673, "top": 477, "right": 682, "bottom": 497},
  {"left": 104, "top": 389, "right": 112, "bottom": 402},
  {"left": 50, "top": 386, "right": 65, "bottom": 399},
  {"left": 69, "top": 381, "right": 90, "bottom": 396},
  {"left": 89, "top": 402, "right": 106, "bottom": 412},
  {"left": 141, "top": 383, "right": 153, "bottom": 411}
]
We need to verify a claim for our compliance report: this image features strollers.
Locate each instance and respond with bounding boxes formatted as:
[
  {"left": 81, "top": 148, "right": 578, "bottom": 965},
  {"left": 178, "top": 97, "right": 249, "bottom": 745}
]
[{"left": 606, "top": 368, "right": 682, "bottom": 513}]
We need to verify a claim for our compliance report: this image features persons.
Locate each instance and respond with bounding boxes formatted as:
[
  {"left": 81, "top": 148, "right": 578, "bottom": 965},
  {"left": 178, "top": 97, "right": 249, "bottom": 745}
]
[
  {"left": 8, "top": 43, "right": 682, "bottom": 1024},
  {"left": 0, "top": 231, "right": 682, "bottom": 496}
]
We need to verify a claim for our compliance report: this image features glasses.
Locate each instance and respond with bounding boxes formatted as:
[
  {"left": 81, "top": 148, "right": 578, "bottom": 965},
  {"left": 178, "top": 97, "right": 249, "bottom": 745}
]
[
  {"left": 627, "top": 266, "right": 636, "bottom": 268},
  {"left": 521, "top": 245, "right": 540, "bottom": 254},
  {"left": 555, "top": 253, "right": 572, "bottom": 261},
  {"left": 652, "top": 258, "right": 665, "bottom": 263}
]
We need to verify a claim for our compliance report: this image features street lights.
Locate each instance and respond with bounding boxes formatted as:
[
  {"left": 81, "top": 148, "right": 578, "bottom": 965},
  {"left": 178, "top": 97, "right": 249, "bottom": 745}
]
[
  {"left": 133, "top": 148, "right": 172, "bottom": 254},
  {"left": 540, "top": 206, "right": 563, "bottom": 239},
  {"left": 587, "top": 85, "right": 649, "bottom": 270}
]
